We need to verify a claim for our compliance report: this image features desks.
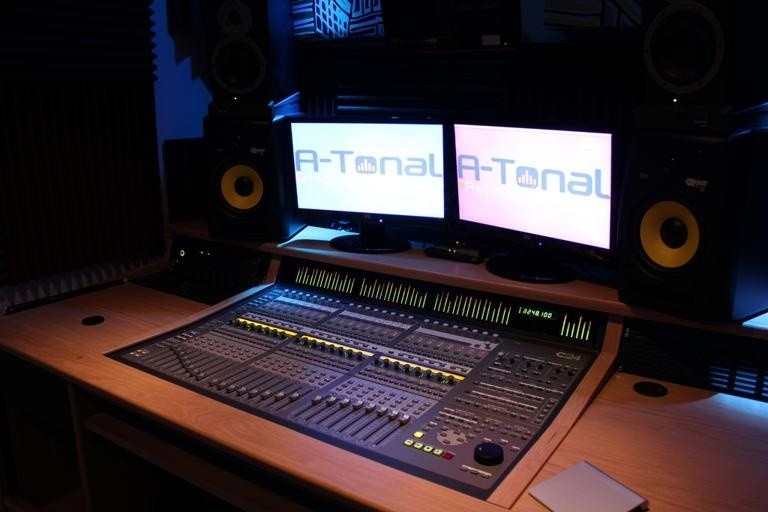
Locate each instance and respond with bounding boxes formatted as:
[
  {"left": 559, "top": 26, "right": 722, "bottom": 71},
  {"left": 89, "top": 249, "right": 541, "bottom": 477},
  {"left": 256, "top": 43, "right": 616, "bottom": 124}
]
[{"left": 2, "top": 225, "right": 768, "bottom": 511}]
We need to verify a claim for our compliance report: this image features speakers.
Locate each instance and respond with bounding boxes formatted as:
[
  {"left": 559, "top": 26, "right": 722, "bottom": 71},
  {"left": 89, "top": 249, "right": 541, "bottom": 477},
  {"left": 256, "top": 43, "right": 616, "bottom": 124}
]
[
  {"left": 617, "top": 96, "right": 768, "bottom": 325},
  {"left": 203, "top": 95, "right": 310, "bottom": 243}
]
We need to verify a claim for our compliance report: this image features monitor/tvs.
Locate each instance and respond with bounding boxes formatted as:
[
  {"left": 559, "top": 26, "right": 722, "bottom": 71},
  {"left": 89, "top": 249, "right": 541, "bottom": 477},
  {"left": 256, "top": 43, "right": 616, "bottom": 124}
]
[
  {"left": 448, "top": 119, "right": 621, "bottom": 284},
  {"left": 283, "top": 114, "right": 452, "bottom": 255}
]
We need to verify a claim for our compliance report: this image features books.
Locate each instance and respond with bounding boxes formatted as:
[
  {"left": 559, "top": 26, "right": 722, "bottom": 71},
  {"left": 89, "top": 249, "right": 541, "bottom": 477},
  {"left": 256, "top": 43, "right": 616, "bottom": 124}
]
[{"left": 527, "top": 461, "right": 649, "bottom": 512}]
